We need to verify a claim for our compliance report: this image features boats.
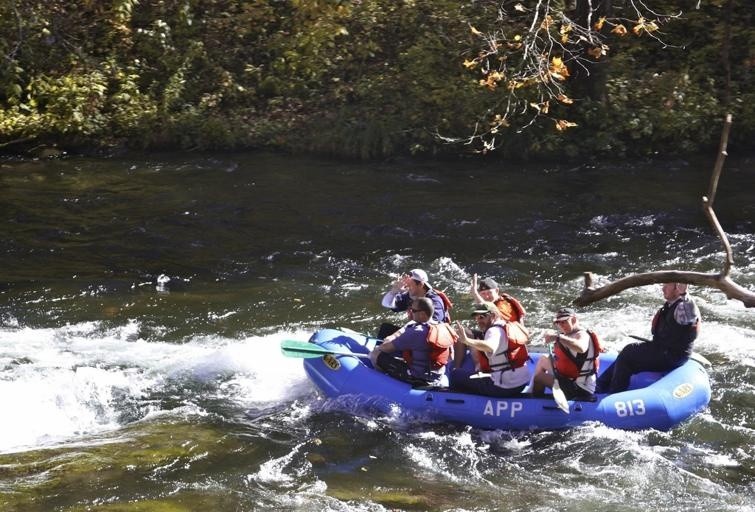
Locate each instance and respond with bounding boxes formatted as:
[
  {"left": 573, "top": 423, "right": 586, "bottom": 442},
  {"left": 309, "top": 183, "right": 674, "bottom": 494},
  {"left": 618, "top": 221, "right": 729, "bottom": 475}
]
[{"left": 301, "top": 326, "right": 715, "bottom": 434}]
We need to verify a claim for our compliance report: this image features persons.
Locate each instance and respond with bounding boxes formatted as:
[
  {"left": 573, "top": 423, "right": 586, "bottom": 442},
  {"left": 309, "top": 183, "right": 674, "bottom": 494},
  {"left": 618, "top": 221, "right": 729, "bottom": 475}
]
[
  {"left": 596, "top": 280, "right": 701, "bottom": 398},
  {"left": 376, "top": 268, "right": 455, "bottom": 345},
  {"left": 451, "top": 300, "right": 531, "bottom": 399},
  {"left": 368, "top": 296, "right": 458, "bottom": 383},
  {"left": 468, "top": 271, "right": 527, "bottom": 327},
  {"left": 531, "top": 307, "right": 604, "bottom": 402}
]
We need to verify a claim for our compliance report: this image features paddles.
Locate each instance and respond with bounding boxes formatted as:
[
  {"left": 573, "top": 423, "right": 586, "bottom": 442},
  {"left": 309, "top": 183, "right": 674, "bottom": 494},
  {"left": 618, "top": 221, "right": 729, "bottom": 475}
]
[
  {"left": 548, "top": 342, "right": 569, "bottom": 414},
  {"left": 281, "top": 340, "right": 404, "bottom": 361}
]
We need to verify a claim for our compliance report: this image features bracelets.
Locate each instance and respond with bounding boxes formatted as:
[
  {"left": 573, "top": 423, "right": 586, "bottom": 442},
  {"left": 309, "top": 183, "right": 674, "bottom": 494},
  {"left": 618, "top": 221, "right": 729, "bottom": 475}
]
[
  {"left": 555, "top": 334, "right": 561, "bottom": 342},
  {"left": 679, "top": 292, "right": 687, "bottom": 296}
]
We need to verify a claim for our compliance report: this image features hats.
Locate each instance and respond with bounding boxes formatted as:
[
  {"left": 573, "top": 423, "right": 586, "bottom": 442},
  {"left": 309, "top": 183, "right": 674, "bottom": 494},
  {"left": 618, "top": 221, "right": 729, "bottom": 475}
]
[
  {"left": 407, "top": 268, "right": 432, "bottom": 289},
  {"left": 478, "top": 276, "right": 497, "bottom": 292},
  {"left": 553, "top": 308, "right": 575, "bottom": 324},
  {"left": 470, "top": 301, "right": 498, "bottom": 317}
]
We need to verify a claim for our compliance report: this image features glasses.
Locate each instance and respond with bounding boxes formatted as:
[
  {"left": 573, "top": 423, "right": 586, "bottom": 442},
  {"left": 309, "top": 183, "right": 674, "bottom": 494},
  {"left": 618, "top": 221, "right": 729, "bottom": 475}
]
[
  {"left": 474, "top": 313, "right": 490, "bottom": 322},
  {"left": 411, "top": 309, "right": 421, "bottom": 313}
]
